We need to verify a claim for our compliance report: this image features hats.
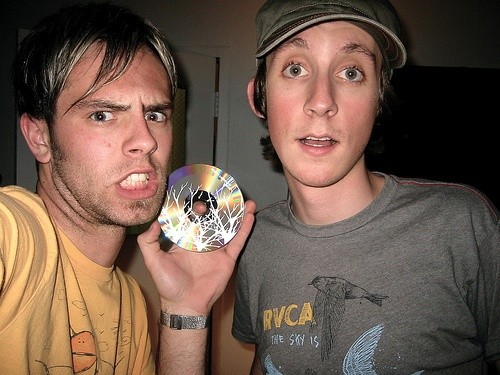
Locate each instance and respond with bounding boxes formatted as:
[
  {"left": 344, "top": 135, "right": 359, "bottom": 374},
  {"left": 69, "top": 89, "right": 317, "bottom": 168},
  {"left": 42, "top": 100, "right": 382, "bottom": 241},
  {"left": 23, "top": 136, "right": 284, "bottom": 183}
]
[{"left": 255, "top": 0, "right": 406, "bottom": 72}]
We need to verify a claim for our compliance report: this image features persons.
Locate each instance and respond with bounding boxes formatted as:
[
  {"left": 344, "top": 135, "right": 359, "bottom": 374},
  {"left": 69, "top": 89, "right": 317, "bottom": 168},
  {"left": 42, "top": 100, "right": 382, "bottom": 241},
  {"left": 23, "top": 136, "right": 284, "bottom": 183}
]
[
  {"left": 0, "top": 7, "right": 256, "bottom": 375},
  {"left": 231, "top": 0, "right": 500, "bottom": 375}
]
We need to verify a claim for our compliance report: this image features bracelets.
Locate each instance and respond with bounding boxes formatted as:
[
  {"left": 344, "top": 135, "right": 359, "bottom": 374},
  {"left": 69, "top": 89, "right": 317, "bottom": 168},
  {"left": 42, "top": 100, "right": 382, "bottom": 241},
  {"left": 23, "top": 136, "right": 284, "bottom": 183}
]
[{"left": 158, "top": 310, "right": 210, "bottom": 330}]
